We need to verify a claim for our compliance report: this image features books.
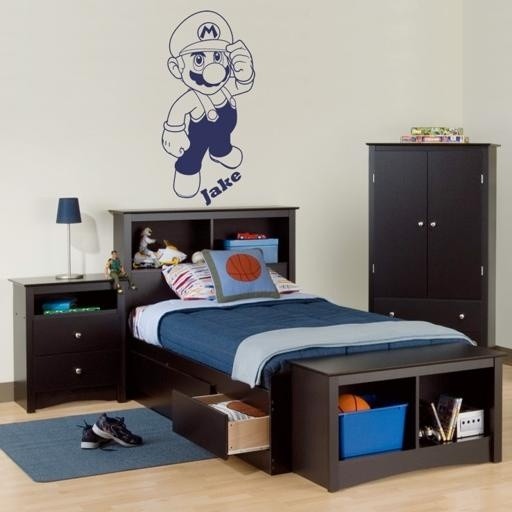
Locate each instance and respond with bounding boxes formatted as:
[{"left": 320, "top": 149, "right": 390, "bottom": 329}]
[
  {"left": 420, "top": 394, "right": 464, "bottom": 442},
  {"left": 400, "top": 127, "right": 468, "bottom": 144}
]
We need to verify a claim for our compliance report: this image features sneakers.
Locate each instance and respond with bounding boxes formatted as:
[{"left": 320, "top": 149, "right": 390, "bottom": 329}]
[
  {"left": 80, "top": 424, "right": 112, "bottom": 450},
  {"left": 90, "top": 412, "right": 144, "bottom": 447}
]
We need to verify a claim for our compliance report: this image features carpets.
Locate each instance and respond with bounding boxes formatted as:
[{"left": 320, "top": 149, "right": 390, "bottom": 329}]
[{"left": 0, "top": 407, "right": 229, "bottom": 483}]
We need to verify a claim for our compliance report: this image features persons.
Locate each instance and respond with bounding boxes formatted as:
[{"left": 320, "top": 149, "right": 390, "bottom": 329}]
[
  {"left": 133, "top": 227, "right": 187, "bottom": 268},
  {"left": 104, "top": 251, "right": 137, "bottom": 294}
]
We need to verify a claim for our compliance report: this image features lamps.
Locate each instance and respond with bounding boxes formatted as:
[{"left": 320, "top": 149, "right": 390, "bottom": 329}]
[{"left": 54, "top": 197, "right": 82, "bottom": 279}]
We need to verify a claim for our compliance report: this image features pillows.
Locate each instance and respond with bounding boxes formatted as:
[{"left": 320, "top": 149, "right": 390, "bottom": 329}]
[
  {"left": 202, "top": 248, "right": 281, "bottom": 304},
  {"left": 161, "top": 261, "right": 303, "bottom": 302}
]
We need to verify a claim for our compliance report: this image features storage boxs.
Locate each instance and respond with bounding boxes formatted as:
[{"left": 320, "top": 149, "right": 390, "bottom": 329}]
[
  {"left": 338, "top": 404, "right": 408, "bottom": 459},
  {"left": 224, "top": 236, "right": 280, "bottom": 266},
  {"left": 454, "top": 408, "right": 486, "bottom": 439}
]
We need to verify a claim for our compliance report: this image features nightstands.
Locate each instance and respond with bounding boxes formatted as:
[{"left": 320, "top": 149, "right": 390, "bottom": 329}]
[{"left": 8, "top": 273, "right": 129, "bottom": 414}]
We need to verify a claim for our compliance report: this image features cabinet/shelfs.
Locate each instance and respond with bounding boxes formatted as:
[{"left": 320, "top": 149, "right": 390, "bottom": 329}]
[
  {"left": 366, "top": 144, "right": 499, "bottom": 301},
  {"left": 369, "top": 296, "right": 496, "bottom": 359}
]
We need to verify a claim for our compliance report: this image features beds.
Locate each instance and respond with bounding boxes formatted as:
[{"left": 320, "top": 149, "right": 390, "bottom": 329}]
[{"left": 107, "top": 205, "right": 511, "bottom": 493}]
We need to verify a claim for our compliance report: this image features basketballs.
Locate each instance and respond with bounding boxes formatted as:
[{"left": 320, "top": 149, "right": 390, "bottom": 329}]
[
  {"left": 226, "top": 253, "right": 261, "bottom": 281},
  {"left": 338, "top": 392, "right": 369, "bottom": 414}
]
